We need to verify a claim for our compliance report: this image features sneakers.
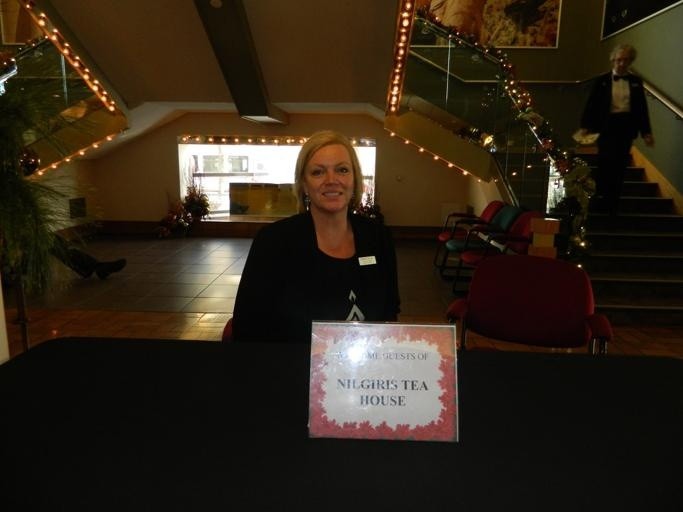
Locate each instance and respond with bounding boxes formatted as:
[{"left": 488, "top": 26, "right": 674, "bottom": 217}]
[{"left": 94, "top": 257, "right": 126, "bottom": 280}]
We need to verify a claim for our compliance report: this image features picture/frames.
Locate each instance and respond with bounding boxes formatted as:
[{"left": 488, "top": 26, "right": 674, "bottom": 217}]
[
  {"left": 407, "top": 0, "right": 563, "bottom": 50},
  {"left": 599, "top": 0, "right": 683, "bottom": 42}
]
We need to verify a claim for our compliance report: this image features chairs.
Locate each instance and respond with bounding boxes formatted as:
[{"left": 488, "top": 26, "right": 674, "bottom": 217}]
[{"left": 432, "top": 201, "right": 613, "bottom": 357}]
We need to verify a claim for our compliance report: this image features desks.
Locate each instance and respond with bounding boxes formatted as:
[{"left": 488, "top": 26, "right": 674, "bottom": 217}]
[{"left": 0, "top": 337, "right": 683, "bottom": 512}]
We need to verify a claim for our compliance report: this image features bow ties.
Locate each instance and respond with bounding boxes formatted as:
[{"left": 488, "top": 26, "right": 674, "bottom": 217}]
[{"left": 613, "top": 75, "right": 630, "bottom": 82}]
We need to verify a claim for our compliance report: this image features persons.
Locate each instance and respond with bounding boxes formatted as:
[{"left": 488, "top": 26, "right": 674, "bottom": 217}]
[
  {"left": 230, "top": 129, "right": 404, "bottom": 343},
  {"left": 49, "top": 233, "right": 130, "bottom": 280},
  {"left": 575, "top": 42, "right": 656, "bottom": 216}
]
